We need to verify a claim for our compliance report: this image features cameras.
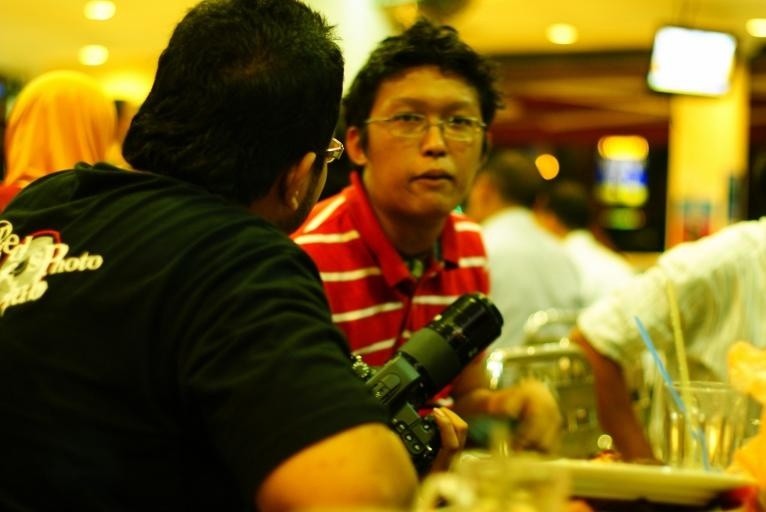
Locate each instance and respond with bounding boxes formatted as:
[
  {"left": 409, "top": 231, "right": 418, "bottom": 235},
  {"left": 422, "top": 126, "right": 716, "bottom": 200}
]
[{"left": 350, "top": 291, "right": 503, "bottom": 476}]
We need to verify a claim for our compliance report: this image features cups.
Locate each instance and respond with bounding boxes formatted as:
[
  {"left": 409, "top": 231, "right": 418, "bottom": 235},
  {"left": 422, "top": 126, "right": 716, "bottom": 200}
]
[{"left": 664, "top": 381, "right": 752, "bottom": 468}]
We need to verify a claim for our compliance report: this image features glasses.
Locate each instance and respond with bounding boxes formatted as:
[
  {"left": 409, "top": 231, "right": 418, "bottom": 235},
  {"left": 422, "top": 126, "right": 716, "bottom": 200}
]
[
  {"left": 362, "top": 111, "right": 488, "bottom": 143},
  {"left": 324, "top": 138, "right": 344, "bottom": 163}
]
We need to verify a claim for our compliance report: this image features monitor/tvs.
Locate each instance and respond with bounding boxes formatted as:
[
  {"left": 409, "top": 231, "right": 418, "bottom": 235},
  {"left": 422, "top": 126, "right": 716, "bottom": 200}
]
[{"left": 646, "top": 25, "right": 739, "bottom": 100}]
